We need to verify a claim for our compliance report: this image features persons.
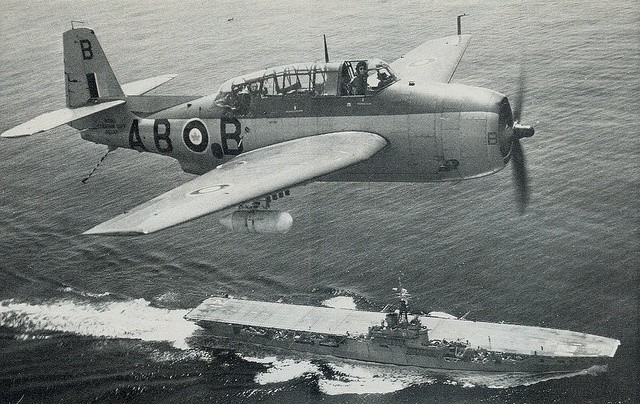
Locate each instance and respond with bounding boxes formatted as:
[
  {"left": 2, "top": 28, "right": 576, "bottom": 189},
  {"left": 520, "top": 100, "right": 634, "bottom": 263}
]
[{"left": 348, "top": 60, "right": 368, "bottom": 96}]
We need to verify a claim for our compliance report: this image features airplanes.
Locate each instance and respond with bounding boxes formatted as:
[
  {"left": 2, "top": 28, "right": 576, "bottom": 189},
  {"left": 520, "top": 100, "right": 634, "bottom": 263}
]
[{"left": 0, "top": 12, "right": 534, "bottom": 237}]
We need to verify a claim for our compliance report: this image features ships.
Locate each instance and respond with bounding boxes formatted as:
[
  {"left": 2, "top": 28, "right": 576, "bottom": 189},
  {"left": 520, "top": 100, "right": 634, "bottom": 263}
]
[{"left": 183, "top": 294, "right": 622, "bottom": 373}]
